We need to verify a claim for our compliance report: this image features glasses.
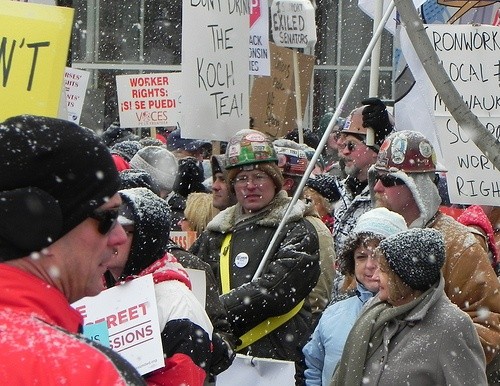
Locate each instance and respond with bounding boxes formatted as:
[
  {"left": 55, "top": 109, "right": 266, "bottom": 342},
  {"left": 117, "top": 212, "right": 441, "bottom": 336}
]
[
  {"left": 89, "top": 206, "right": 122, "bottom": 236},
  {"left": 373, "top": 170, "right": 405, "bottom": 187},
  {"left": 344, "top": 140, "right": 355, "bottom": 152},
  {"left": 233, "top": 173, "right": 268, "bottom": 184}
]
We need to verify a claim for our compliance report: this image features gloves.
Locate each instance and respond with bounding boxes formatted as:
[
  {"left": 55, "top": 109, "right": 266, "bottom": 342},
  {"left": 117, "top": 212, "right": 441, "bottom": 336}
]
[
  {"left": 361, "top": 97, "right": 394, "bottom": 144},
  {"left": 208, "top": 329, "right": 242, "bottom": 376}
]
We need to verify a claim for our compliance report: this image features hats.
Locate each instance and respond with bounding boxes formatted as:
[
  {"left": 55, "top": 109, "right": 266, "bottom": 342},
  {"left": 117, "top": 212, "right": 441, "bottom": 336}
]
[
  {"left": 167, "top": 129, "right": 196, "bottom": 150},
  {"left": 129, "top": 146, "right": 178, "bottom": 189},
  {"left": 272, "top": 128, "right": 326, "bottom": 171},
  {"left": 176, "top": 156, "right": 205, "bottom": 182},
  {"left": 305, "top": 174, "right": 341, "bottom": 202},
  {"left": 111, "top": 140, "right": 142, "bottom": 161},
  {"left": 379, "top": 227, "right": 446, "bottom": 291},
  {"left": 0, "top": 115, "right": 123, "bottom": 263},
  {"left": 137, "top": 137, "right": 164, "bottom": 150},
  {"left": 191, "top": 139, "right": 213, "bottom": 156},
  {"left": 353, "top": 206, "right": 408, "bottom": 240}
]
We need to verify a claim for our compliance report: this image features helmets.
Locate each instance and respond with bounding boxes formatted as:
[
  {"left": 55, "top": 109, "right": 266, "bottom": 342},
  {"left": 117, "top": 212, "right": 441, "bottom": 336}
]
[
  {"left": 374, "top": 130, "right": 449, "bottom": 173},
  {"left": 223, "top": 129, "right": 278, "bottom": 170},
  {"left": 340, "top": 105, "right": 368, "bottom": 135}
]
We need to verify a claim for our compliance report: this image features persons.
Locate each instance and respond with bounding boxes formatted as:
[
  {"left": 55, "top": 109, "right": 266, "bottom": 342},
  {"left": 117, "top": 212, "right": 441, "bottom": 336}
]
[
  {"left": 188, "top": 129, "right": 320, "bottom": 386},
  {"left": 102, "top": 98, "right": 500, "bottom": 386},
  {"left": 0, "top": 114, "right": 237, "bottom": 386},
  {"left": 329, "top": 228, "right": 488, "bottom": 386},
  {"left": 102, "top": 188, "right": 212, "bottom": 386}
]
